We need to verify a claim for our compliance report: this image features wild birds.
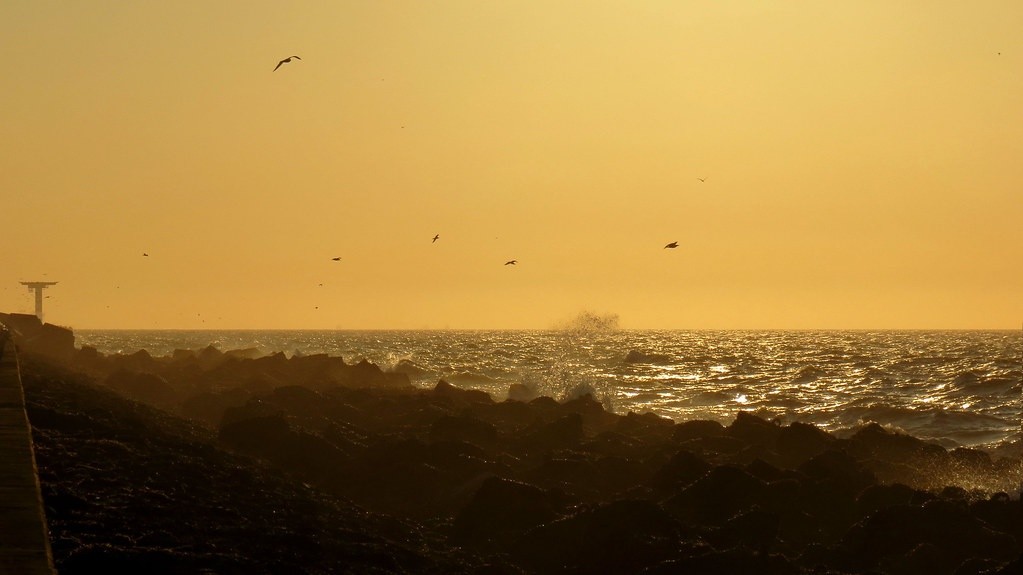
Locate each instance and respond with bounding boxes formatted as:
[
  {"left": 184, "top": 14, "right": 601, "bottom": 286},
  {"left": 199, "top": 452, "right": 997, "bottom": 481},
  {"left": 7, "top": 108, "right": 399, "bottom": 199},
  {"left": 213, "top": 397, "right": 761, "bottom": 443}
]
[
  {"left": 665, "top": 241, "right": 679, "bottom": 249},
  {"left": 505, "top": 259, "right": 519, "bottom": 266},
  {"left": 332, "top": 256, "right": 342, "bottom": 262},
  {"left": 432, "top": 233, "right": 440, "bottom": 243},
  {"left": 271, "top": 54, "right": 303, "bottom": 71}
]
